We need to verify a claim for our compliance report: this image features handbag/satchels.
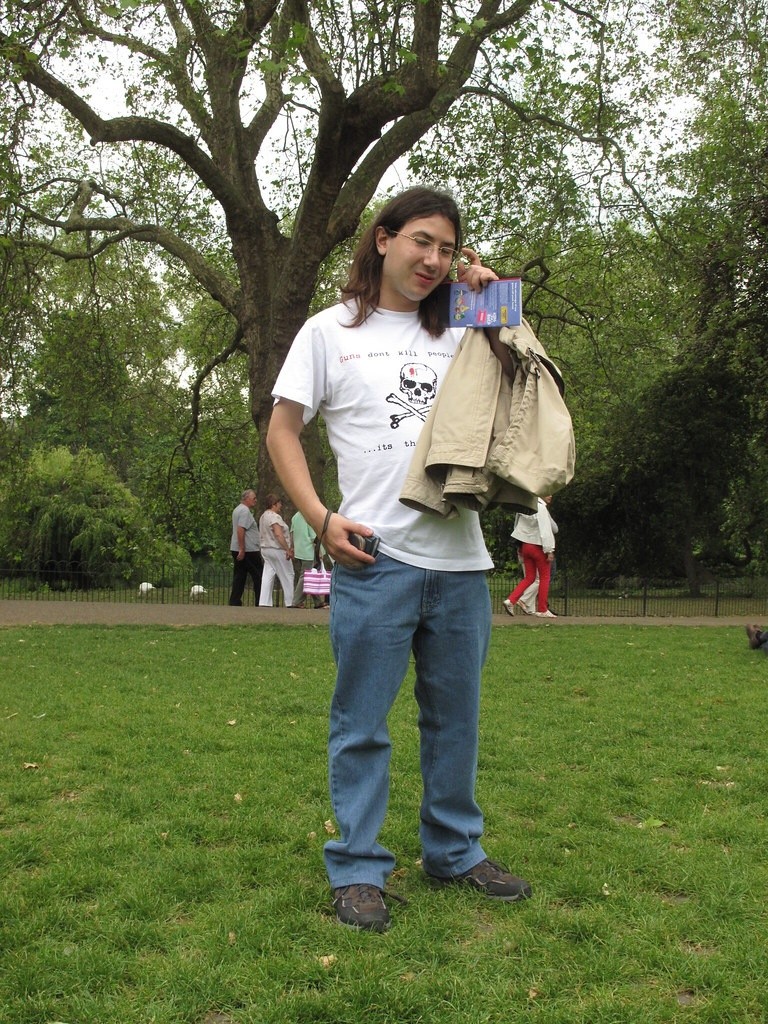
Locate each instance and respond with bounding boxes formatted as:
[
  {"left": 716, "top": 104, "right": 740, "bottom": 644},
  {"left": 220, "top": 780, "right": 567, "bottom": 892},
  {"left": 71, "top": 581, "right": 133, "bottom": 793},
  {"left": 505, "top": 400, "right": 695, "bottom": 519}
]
[{"left": 303, "top": 557, "right": 332, "bottom": 595}]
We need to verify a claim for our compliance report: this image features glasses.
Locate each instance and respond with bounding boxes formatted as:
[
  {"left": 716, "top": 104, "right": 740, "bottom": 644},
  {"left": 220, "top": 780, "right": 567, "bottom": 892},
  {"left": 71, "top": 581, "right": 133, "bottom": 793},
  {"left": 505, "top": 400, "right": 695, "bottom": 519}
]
[{"left": 388, "top": 229, "right": 461, "bottom": 264}]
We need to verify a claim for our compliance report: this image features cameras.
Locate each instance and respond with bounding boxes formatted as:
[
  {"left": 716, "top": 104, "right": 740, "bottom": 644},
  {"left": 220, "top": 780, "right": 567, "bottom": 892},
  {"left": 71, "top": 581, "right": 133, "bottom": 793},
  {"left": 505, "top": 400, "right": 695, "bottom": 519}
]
[{"left": 348, "top": 531, "right": 380, "bottom": 556}]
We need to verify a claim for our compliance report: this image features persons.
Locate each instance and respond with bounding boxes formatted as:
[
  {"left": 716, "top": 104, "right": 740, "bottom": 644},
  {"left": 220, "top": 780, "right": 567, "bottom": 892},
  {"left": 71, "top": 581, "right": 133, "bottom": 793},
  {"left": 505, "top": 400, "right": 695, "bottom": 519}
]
[
  {"left": 259, "top": 494, "right": 295, "bottom": 608},
  {"left": 265, "top": 189, "right": 532, "bottom": 934},
  {"left": 229, "top": 489, "right": 264, "bottom": 607},
  {"left": 502, "top": 494, "right": 558, "bottom": 617},
  {"left": 290, "top": 509, "right": 330, "bottom": 609}
]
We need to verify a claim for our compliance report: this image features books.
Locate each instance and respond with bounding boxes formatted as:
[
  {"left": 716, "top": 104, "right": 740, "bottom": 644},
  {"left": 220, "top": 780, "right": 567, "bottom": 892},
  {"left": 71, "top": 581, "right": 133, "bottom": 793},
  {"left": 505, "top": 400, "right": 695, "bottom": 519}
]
[{"left": 449, "top": 278, "right": 522, "bottom": 328}]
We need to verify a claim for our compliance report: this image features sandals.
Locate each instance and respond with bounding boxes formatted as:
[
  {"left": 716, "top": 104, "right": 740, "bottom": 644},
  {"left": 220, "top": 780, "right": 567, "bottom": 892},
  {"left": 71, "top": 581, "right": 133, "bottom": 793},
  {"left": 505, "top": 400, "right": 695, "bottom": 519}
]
[{"left": 516, "top": 600, "right": 535, "bottom": 615}]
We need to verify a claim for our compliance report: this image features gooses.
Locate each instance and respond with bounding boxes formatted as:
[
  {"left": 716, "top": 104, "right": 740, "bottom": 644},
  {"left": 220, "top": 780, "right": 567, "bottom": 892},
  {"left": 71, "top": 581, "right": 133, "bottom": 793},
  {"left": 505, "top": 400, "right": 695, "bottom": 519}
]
[
  {"left": 188, "top": 584, "right": 209, "bottom": 602},
  {"left": 139, "top": 581, "right": 157, "bottom": 600}
]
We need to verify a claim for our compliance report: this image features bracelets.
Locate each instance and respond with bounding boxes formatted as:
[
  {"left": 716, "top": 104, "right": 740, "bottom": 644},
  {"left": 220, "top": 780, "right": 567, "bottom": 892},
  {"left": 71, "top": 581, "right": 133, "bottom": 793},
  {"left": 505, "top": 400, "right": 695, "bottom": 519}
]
[{"left": 312, "top": 509, "right": 333, "bottom": 570}]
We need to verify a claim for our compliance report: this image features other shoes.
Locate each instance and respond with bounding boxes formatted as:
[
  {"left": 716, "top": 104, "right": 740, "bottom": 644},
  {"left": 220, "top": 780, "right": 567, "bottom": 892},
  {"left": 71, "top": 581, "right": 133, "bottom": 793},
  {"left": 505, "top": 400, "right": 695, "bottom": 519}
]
[
  {"left": 287, "top": 604, "right": 305, "bottom": 608},
  {"left": 314, "top": 602, "right": 328, "bottom": 609},
  {"left": 746, "top": 623, "right": 761, "bottom": 649}
]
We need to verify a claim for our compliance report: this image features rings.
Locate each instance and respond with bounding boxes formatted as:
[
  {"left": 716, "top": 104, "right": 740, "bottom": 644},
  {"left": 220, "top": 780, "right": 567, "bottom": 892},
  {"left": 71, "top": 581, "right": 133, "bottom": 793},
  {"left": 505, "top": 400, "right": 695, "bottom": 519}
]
[{"left": 239, "top": 557, "right": 240, "bottom": 559}]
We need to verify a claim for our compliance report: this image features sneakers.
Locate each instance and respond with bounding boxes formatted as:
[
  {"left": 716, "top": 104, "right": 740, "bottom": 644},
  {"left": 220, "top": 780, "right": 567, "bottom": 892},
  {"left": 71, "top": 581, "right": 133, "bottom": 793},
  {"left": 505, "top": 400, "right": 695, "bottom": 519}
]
[
  {"left": 427, "top": 858, "right": 532, "bottom": 902},
  {"left": 503, "top": 598, "right": 514, "bottom": 616},
  {"left": 332, "top": 883, "right": 406, "bottom": 927},
  {"left": 537, "top": 610, "right": 557, "bottom": 618}
]
